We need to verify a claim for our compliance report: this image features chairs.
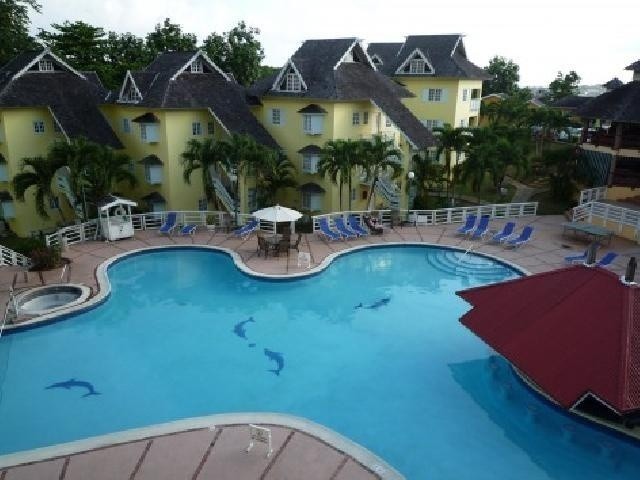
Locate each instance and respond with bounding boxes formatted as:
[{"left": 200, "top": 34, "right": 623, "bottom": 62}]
[
  {"left": 318, "top": 215, "right": 383, "bottom": 242},
  {"left": 256, "top": 228, "right": 303, "bottom": 259},
  {"left": 565, "top": 243, "right": 618, "bottom": 268},
  {"left": 456, "top": 214, "right": 533, "bottom": 250},
  {"left": 159, "top": 213, "right": 197, "bottom": 240},
  {"left": 233, "top": 221, "right": 260, "bottom": 238}
]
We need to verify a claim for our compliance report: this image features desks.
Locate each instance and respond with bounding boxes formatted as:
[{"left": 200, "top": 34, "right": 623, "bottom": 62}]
[{"left": 562, "top": 221, "right": 614, "bottom": 245}]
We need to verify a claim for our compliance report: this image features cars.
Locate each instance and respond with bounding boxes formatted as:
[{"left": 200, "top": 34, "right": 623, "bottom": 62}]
[{"left": 531, "top": 126, "right": 595, "bottom": 141}]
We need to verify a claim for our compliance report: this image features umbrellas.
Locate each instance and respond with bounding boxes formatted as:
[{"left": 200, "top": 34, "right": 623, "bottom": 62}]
[{"left": 250, "top": 203, "right": 305, "bottom": 234}]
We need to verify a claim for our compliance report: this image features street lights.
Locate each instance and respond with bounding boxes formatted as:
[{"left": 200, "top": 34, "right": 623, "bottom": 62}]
[
  {"left": 408, "top": 169, "right": 415, "bottom": 210},
  {"left": 229, "top": 162, "right": 238, "bottom": 226}
]
